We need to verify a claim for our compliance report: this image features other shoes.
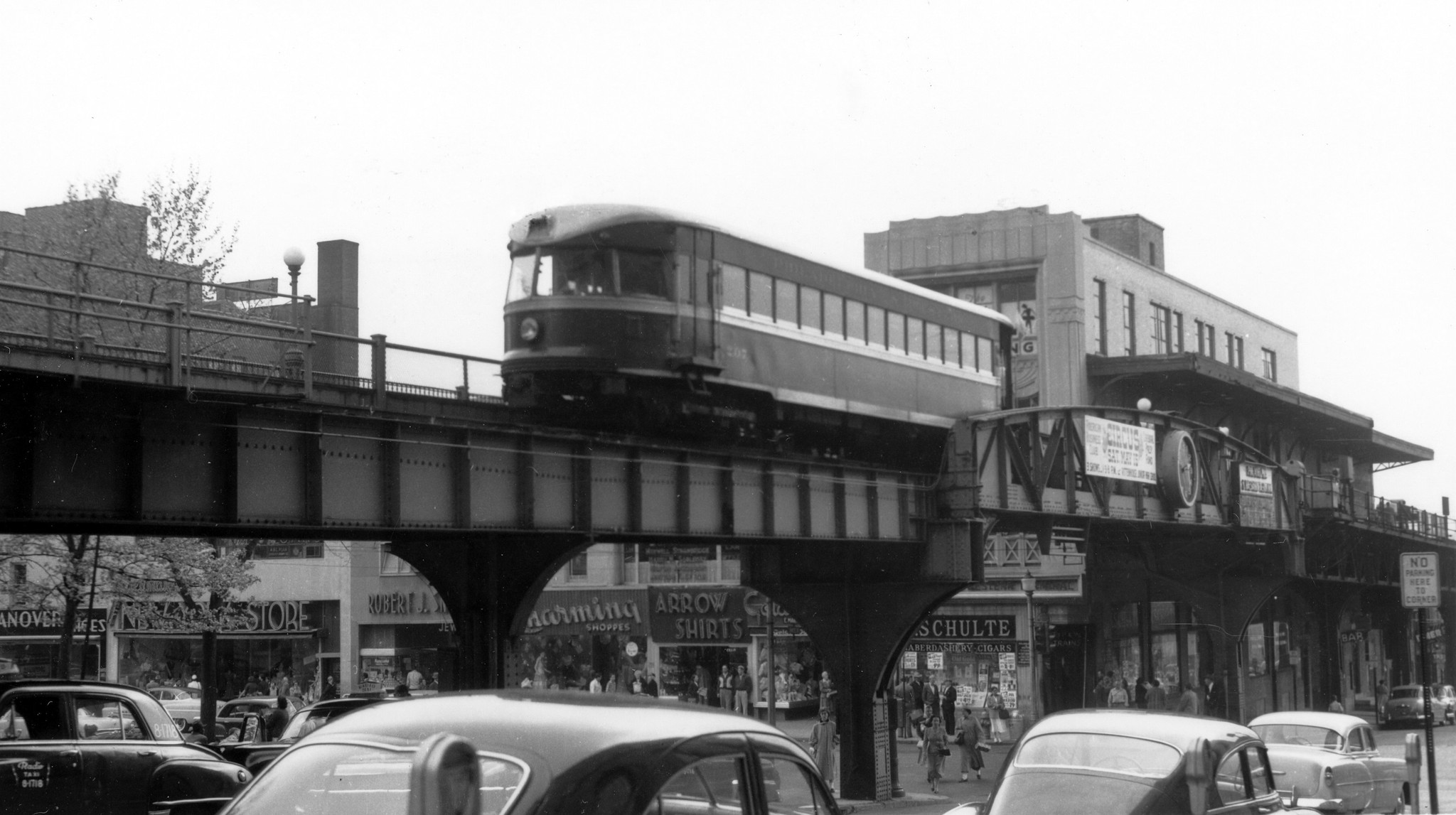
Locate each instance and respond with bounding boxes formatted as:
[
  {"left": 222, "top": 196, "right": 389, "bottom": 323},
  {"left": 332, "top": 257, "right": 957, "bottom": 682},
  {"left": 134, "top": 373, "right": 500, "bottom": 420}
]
[
  {"left": 935, "top": 791, "right": 939, "bottom": 794},
  {"left": 931, "top": 788, "right": 934, "bottom": 791},
  {"left": 994, "top": 738, "right": 998, "bottom": 742},
  {"left": 959, "top": 778, "right": 968, "bottom": 782},
  {"left": 999, "top": 738, "right": 1001, "bottom": 743},
  {"left": 977, "top": 774, "right": 981, "bottom": 779},
  {"left": 830, "top": 789, "right": 835, "bottom": 793}
]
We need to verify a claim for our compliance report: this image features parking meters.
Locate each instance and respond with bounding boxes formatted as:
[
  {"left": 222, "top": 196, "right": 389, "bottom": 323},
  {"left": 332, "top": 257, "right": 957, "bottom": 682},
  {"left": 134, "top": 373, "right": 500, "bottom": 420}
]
[{"left": 1404, "top": 725, "right": 1440, "bottom": 815}]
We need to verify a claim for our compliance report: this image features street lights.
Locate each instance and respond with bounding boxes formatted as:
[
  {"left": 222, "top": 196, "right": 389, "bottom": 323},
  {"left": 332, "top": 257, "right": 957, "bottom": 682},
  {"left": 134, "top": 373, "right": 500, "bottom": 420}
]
[
  {"left": 1021, "top": 570, "right": 1040, "bottom": 720},
  {"left": 279, "top": 247, "right": 309, "bottom": 398}
]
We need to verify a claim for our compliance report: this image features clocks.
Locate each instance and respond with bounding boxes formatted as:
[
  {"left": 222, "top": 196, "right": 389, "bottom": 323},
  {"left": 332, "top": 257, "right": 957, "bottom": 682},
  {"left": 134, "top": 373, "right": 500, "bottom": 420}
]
[{"left": 1161, "top": 430, "right": 1203, "bottom": 509}]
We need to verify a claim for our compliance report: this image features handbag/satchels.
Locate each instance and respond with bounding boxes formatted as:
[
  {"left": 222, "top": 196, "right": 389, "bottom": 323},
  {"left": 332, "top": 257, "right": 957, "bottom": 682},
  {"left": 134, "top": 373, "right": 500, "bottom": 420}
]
[
  {"left": 916, "top": 740, "right": 924, "bottom": 749},
  {"left": 999, "top": 703, "right": 1010, "bottom": 719},
  {"left": 954, "top": 733, "right": 964, "bottom": 745},
  {"left": 976, "top": 743, "right": 992, "bottom": 753},
  {"left": 938, "top": 749, "right": 950, "bottom": 756}
]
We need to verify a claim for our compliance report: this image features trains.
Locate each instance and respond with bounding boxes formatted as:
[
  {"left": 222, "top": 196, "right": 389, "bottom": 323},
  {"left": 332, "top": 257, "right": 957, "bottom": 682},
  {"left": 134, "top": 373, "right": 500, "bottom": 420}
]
[{"left": 496, "top": 200, "right": 1016, "bottom": 472}]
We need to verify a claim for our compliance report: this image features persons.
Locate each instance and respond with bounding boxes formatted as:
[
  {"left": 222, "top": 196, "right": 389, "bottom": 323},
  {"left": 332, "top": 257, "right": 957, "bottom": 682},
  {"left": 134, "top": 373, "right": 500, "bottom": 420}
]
[
  {"left": 1092, "top": 658, "right": 1281, "bottom": 716},
  {"left": 120, "top": 651, "right": 1008, "bottom": 794},
  {"left": 1329, "top": 694, "right": 1344, "bottom": 714},
  {"left": 1376, "top": 679, "right": 1388, "bottom": 712}
]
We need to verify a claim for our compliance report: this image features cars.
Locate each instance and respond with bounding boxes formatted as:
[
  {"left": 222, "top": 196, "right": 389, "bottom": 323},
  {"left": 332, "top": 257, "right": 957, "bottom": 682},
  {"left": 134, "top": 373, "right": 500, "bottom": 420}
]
[
  {"left": 216, "top": 695, "right": 308, "bottom": 735},
  {"left": 0, "top": 679, "right": 255, "bottom": 815},
  {"left": 1217, "top": 711, "right": 1413, "bottom": 815},
  {"left": 147, "top": 686, "right": 228, "bottom": 734},
  {"left": 1379, "top": 683, "right": 1456, "bottom": 729},
  {"left": 219, "top": 688, "right": 854, "bottom": 815},
  {"left": 941, "top": 707, "right": 1322, "bottom": 815},
  {"left": 1, "top": 701, "right": 136, "bottom": 741},
  {"left": 229, "top": 694, "right": 406, "bottom": 776}
]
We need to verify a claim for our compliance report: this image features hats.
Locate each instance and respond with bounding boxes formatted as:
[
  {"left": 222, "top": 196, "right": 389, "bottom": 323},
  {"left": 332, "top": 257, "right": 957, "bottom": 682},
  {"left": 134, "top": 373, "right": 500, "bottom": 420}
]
[
  {"left": 913, "top": 671, "right": 922, "bottom": 677},
  {"left": 1204, "top": 674, "right": 1213, "bottom": 681}
]
